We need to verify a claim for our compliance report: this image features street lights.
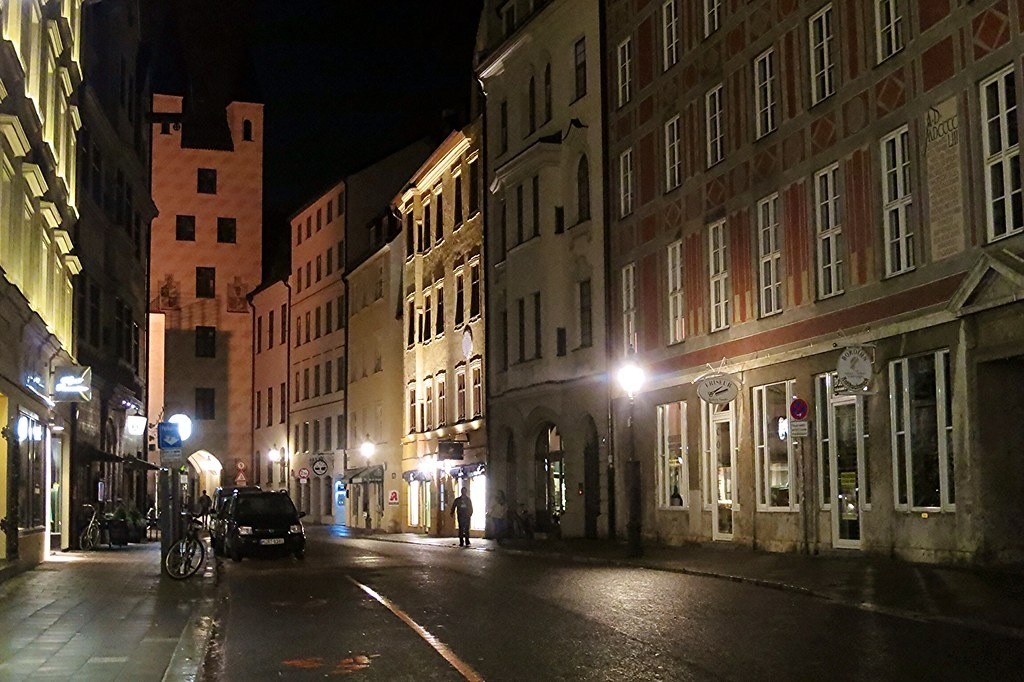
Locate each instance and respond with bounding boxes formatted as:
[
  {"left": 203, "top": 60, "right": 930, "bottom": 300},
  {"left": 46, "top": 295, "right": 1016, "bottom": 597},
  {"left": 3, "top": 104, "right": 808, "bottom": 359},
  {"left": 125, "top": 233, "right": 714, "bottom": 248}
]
[
  {"left": 360, "top": 433, "right": 375, "bottom": 530},
  {"left": 622, "top": 345, "right": 645, "bottom": 557}
]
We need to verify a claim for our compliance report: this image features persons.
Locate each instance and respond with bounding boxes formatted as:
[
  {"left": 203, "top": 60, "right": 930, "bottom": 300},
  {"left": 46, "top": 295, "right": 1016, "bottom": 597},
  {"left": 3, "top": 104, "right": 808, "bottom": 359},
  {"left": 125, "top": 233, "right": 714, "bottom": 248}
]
[
  {"left": 487, "top": 489, "right": 509, "bottom": 547},
  {"left": 198, "top": 490, "right": 213, "bottom": 531},
  {"left": 449, "top": 486, "right": 474, "bottom": 548}
]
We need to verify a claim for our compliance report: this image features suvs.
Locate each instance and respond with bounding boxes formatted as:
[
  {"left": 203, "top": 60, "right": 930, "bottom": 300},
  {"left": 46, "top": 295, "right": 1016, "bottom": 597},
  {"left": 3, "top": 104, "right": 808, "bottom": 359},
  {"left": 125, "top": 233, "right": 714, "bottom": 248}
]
[
  {"left": 222, "top": 489, "right": 307, "bottom": 561},
  {"left": 208, "top": 486, "right": 263, "bottom": 547}
]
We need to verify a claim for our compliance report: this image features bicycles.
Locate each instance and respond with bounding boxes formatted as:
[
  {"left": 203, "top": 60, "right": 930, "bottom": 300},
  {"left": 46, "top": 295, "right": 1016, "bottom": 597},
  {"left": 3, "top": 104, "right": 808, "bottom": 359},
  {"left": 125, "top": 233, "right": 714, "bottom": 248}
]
[
  {"left": 165, "top": 503, "right": 216, "bottom": 580},
  {"left": 145, "top": 508, "right": 163, "bottom": 529},
  {"left": 80, "top": 503, "right": 111, "bottom": 552}
]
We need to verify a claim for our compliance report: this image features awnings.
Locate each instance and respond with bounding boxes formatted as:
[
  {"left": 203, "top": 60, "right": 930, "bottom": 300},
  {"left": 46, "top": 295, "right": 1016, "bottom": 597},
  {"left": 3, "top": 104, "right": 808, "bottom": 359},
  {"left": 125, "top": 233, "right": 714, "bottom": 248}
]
[
  {"left": 74, "top": 440, "right": 171, "bottom": 476},
  {"left": 341, "top": 463, "right": 387, "bottom": 486},
  {"left": 397, "top": 463, "right": 485, "bottom": 487}
]
